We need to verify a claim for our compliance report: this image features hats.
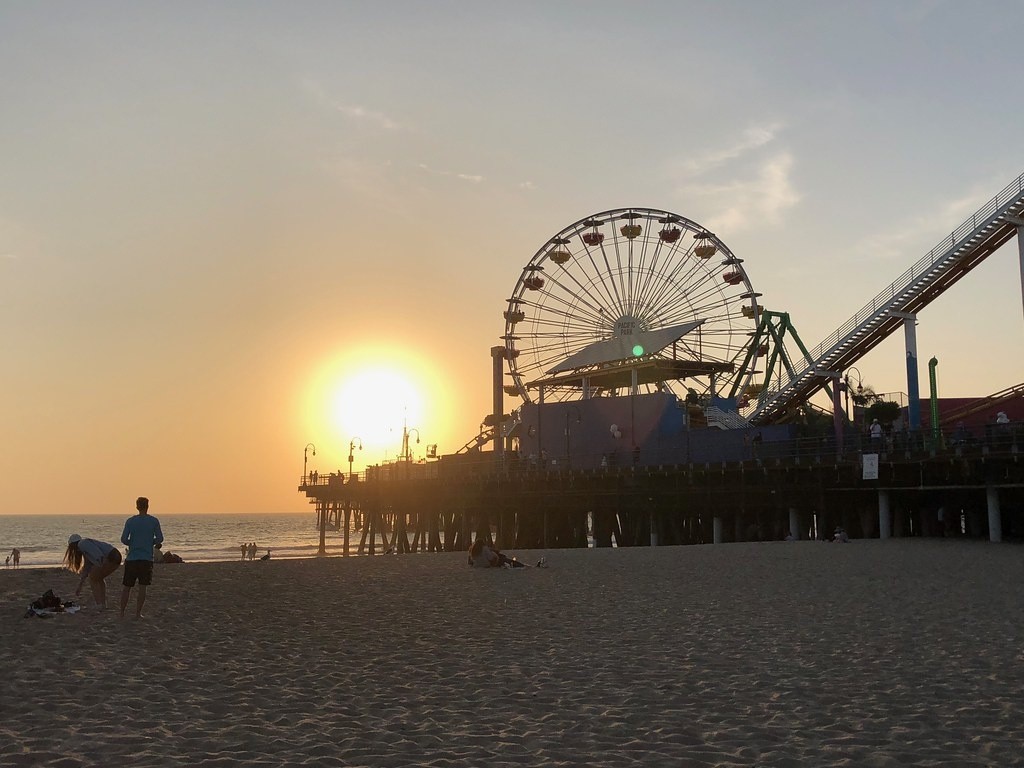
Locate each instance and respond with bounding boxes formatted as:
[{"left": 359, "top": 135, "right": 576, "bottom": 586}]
[{"left": 69, "top": 534, "right": 82, "bottom": 545}]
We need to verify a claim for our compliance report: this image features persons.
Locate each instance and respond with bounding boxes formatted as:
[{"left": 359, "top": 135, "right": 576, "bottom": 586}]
[
  {"left": 469, "top": 538, "right": 531, "bottom": 568},
  {"left": 6, "top": 556, "right": 11, "bottom": 568},
  {"left": 119, "top": 497, "right": 163, "bottom": 618},
  {"left": 687, "top": 388, "right": 698, "bottom": 404},
  {"left": 518, "top": 309, "right": 524, "bottom": 315},
  {"left": 153, "top": 544, "right": 163, "bottom": 563},
  {"left": 309, "top": 470, "right": 318, "bottom": 485},
  {"left": 62, "top": 534, "right": 122, "bottom": 610},
  {"left": 375, "top": 464, "right": 380, "bottom": 480},
  {"left": 11, "top": 548, "right": 20, "bottom": 569},
  {"left": 744, "top": 432, "right": 762, "bottom": 445},
  {"left": 792, "top": 418, "right": 969, "bottom": 461},
  {"left": 240, "top": 542, "right": 270, "bottom": 561},
  {"left": 330, "top": 470, "right": 344, "bottom": 483},
  {"left": 389, "top": 464, "right": 398, "bottom": 481},
  {"left": 124, "top": 548, "right": 129, "bottom": 555},
  {"left": 517, "top": 448, "right": 548, "bottom": 470},
  {"left": 672, "top": 226, "right": 677, "bottom": 230},
  {"left": 834, "top": 527, "right": 848, "bottom": 543}
]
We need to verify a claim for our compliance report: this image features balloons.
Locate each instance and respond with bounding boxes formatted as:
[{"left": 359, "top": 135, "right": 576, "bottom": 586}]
[{"left": 610, "top": 424, "right": 622, "bottom": 439}]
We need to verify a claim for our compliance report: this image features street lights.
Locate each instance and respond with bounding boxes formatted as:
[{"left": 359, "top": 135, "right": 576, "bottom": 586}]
[
  {"left": 406, "top": 428, "right": 419, "bottom": 480},
  {"left": 303, "top": 443, "right": 315, "bottom": 486},
  {"left": 845, "top": 366, "right": 863, "bottom": 464},
  {"left": 348, "top": 437, "right": 362, "bottom": 483}
]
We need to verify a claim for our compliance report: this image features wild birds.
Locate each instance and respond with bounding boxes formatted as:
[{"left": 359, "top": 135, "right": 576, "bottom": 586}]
[
  {"left": 257, "top": 550, "right": 271, "bottom": 565},
  {"left": 382, "top": 545, "right": 395, "bottom": 557}
]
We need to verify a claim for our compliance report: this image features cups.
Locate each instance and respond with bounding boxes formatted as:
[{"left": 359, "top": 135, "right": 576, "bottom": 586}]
[{"left": 537, "top": 557, "right": 548, "bottom": 568}]
